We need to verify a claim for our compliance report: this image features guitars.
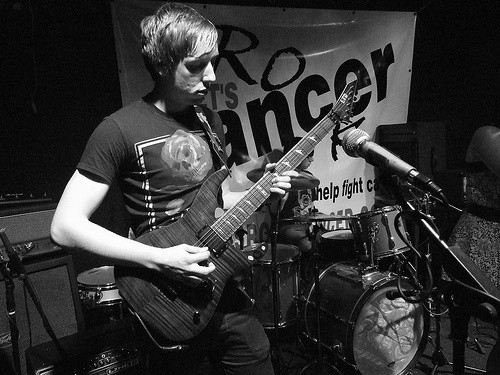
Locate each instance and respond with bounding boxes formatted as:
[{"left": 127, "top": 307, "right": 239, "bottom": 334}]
[{"left": 113, "top": 78, "right": 359, "bottom": 343}]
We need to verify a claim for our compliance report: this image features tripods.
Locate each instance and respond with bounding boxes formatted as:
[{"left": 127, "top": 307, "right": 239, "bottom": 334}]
[
  {"left": 269, "top": 221, "right": 347, "bottom": 375},
  {"left": 419, "top": 179, "right": 488, "bottom": 375}
]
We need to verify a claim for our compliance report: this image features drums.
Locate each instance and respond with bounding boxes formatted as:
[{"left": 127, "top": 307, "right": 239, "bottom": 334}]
[
  {"left": 349, "top": 204, "right": 412, "bottom": 260},
  {"left": 242, "top": 242, "right": 302, "bottom": 330},
  {"left": 320, "top": 229, "right": 355, "bottom": 266},
  {"left": 76, "top": 265, "right": 123, "bottom": 307},
  {"left": 304, "top": 261, "right": 429, "bottom": 375}
]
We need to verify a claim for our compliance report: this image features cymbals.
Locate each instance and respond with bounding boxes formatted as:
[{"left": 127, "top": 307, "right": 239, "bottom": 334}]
[
  {"left": 279, "top": 213, "right": 359, "bottom": 222},
  {"left": 246, "top": 168, "right": 320, "bottom": 191}
]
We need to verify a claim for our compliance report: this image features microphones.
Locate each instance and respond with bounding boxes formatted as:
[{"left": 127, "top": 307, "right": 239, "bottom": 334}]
[{"left": 342, "top": 128, "right": 442, "bottom": 195}]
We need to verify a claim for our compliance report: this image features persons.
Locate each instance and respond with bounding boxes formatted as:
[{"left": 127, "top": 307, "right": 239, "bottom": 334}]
[
  {"left": 448, "top": 120, "right": 500, "bottom": 375},
  {"left": 265, "top": 138, "right": 332, "bottom": 283},
  {"left": 51, "top": 4, "right": 302, "bottom": 375}
]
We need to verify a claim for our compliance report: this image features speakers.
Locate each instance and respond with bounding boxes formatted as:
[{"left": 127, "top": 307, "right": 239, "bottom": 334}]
[{"left": 0, "top": 254, "right": 85, "bottom": 375}]
